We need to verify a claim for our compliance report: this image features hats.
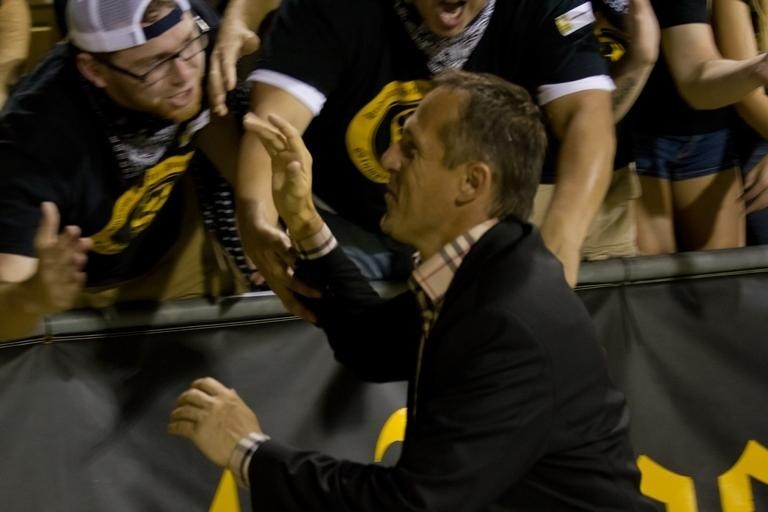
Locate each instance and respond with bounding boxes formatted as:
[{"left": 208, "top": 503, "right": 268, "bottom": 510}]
[{"left": 65, "top": 0, "right": 192, "bottom": 54}]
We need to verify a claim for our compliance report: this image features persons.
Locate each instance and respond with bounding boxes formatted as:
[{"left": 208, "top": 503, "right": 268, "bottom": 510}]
[
  {"left": 166, "top": 74, "right": 661, "bottom": 509},
  {"left": 3, "top": 1, "right": 767, "bottom": 343}
]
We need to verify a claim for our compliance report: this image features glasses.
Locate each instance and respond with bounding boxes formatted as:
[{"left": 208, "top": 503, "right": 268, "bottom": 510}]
[{"left": 88, "top": 8, "right": 212, "bottom": 88}]
